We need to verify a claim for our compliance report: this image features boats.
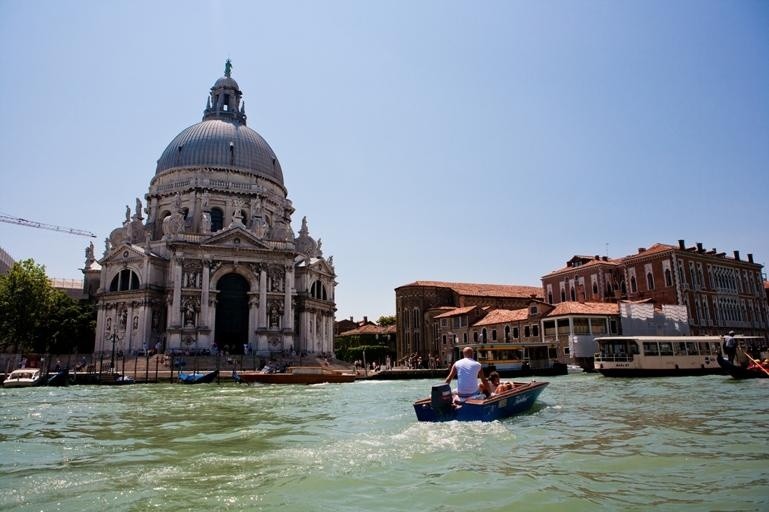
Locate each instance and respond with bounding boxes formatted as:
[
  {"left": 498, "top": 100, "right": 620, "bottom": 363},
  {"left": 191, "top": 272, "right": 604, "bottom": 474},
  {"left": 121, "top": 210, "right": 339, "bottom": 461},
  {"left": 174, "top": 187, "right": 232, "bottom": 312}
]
[
  {"left": 448, "top": 342, "right": 568, "bottom": 378},
  {"left": 46, "top": 368, "right": 69, "bottom": 386},
  {"left": 4, "top": 366, "right": 42, "bottom": 387},
  {"left": 117, "top": 376, "right": 133, "bottom": 384},
  {"left": 592, "top": 335, "right": 768, "bottom": 379},
  {"left": 178, "top": 370, "right": 218, "bottom": 384},
  {"left": 413, "top": 383, "right": 548, "bottom": 421}
]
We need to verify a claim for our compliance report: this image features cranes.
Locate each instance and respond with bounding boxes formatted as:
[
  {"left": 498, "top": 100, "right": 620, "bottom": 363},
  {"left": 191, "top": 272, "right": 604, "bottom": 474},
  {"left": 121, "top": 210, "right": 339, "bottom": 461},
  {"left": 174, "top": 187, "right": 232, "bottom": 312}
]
[{"left": 0, "top": 215, "right": 95, "bottom": 237}]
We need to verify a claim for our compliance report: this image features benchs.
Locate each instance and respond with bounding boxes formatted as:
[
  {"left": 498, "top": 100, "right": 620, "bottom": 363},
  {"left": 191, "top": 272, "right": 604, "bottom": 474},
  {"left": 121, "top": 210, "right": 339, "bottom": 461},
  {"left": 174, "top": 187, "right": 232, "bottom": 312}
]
[{"left": 288, "top": 366, "right": 324, "bottom": 375}]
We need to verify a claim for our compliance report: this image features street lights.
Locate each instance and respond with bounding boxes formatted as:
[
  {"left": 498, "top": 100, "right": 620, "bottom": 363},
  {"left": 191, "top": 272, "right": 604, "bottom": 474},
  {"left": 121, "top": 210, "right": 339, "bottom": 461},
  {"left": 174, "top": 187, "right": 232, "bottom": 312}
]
[{"left": 106, "top": 334, "right": 119, "bottom": 373}]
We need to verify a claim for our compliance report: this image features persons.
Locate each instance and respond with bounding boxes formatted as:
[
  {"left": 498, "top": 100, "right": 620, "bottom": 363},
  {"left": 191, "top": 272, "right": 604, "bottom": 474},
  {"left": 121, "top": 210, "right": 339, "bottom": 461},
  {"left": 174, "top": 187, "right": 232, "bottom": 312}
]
[
  {"left": 725, "top": 330, "right": 739, "bottom": 364},
  {"left": 54, "top": 361, "right": 62, "bottom": 372},
  {"left": 142, "top": 342, "right": 149, "bottom": 356},
  {"left": 19, "top": 357, "right": 30, "bottom": 368},
  {"left": 353, "top": 351, "right": 441, "bottom": 371},
  {"left": 155, "top": 340, "right": 162, "bottom": 354},
  {"left": 444, "top": 346, "right": 491, "bottom": 400},
  {"left": 486, "top": 370, "right": 512, "bottom": 395},
  {"left": 164, "top": 343, "right": 333, "bottom": 380}
]
